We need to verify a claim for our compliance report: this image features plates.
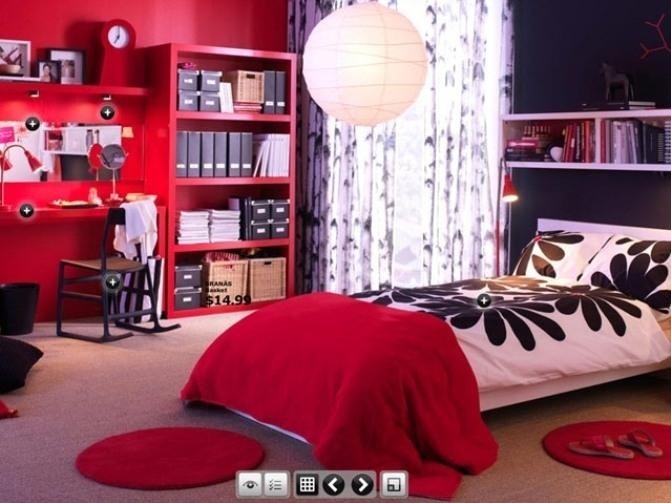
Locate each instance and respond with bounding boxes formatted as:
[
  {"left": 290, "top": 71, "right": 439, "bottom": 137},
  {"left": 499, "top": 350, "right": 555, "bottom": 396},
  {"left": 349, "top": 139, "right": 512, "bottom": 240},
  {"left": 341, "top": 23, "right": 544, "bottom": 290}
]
[{"left": 0, "top": 73, "right": 24, "bottom": 77}]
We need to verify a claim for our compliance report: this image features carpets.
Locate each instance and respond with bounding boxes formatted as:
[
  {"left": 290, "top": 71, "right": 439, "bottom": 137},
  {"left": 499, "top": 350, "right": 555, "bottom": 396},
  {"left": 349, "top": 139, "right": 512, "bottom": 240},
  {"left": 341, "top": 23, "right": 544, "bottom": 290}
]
[
  {"left": 75, "top": 427, "right": 264, "bottom": 490},
  {"left": 540, "top": 418, "right": 671, "bottom": 481}
]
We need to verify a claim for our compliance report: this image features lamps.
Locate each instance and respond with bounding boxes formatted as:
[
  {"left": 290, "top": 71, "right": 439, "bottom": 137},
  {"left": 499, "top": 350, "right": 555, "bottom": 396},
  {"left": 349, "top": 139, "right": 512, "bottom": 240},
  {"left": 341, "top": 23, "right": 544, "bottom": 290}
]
[
  {"left": 302, "top": 0, "right": 429, "bottom": 126},
  {"left": 0, "top": 145, "right": 42, "bottom": 211},
  {"left": 496, "top": 157, "right": 520, "bottom": 277},
  {"left": 0, "top": 150, "right": 13, "bottom": 171}
]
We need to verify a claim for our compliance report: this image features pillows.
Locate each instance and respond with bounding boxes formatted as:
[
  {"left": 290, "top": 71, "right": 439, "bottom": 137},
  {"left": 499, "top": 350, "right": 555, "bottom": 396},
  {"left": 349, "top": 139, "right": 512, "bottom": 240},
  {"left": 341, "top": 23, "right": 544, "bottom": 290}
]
[
  {"left": 578, "top": 233, "right": 671, "bottom": 313},
  {"left": 0, "top": 336, "right": 43, "bottom": 395},
  {"left": 509, "top": 230, "right": 615, "bottom": 284}
]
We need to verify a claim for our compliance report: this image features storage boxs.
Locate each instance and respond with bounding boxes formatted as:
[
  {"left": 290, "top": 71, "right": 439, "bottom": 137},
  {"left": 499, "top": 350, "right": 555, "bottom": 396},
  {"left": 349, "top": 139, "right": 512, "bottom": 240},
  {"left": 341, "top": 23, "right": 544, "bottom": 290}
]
[
  {"left": 175, "top": 256, "right": 286, "bottom": 311},
  {"left": 249, "top": 199, "right": 289, "bottom": 239},
  {"left": 179, "top": 65, "right": 265, "bottom": 113}
]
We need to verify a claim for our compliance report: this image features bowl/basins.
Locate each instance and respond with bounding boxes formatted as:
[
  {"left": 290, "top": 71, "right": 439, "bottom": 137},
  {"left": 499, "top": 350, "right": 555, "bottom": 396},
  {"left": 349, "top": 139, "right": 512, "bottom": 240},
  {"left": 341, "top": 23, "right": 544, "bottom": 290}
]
[{"left": 0, "top": 63, "right": 22, "bottom": 74}]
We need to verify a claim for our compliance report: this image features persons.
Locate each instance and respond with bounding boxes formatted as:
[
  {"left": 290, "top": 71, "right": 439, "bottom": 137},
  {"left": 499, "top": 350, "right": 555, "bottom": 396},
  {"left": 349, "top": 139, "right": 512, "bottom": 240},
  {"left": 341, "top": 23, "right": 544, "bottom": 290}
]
[
  {"left": 41, "top": 64, "right": 55, "bottom": 83},
  {"left": 64, "top": 60, "right": 74, "bottom": 77},
  {"left": 1, "top": 47, "right": 24, "bottom": 69}
]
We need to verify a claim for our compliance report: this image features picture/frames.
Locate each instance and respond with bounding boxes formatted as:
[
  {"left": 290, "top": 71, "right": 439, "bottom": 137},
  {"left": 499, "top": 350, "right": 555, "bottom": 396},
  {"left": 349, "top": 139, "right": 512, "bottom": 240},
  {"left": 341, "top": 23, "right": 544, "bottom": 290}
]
[
  {"left": 36, "top": 60, "right": 62, "bottom": 84},
  {"left": 0, "top": 40, "right": 31, "bottom": 77},
  {"left": 49, "top": 47, "right": 87, "bottom": 85}
]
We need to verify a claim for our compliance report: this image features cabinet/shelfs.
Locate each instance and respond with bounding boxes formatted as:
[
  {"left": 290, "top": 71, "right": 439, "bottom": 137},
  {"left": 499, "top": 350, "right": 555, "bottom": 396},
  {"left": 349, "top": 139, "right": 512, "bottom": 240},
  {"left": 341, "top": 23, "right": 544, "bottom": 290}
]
[
  {"left": 0, "top": 80, "right": 167, "bottom": 317},
  {"left": 502, "top": 109, "right": 671, "bottom": 172},
  {"left": 146, "top": 42, "right": 296, "bottom": 319}
]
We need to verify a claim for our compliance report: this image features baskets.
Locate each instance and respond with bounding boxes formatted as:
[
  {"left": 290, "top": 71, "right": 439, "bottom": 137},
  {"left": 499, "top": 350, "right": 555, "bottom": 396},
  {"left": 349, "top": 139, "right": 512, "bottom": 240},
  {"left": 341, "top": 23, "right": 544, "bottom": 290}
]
[
  {"left": 201, "top": 259, "right": 249, "bottom": 307},
  {"left": 247, "top": 258, "right": 287, "bottom": 303}
]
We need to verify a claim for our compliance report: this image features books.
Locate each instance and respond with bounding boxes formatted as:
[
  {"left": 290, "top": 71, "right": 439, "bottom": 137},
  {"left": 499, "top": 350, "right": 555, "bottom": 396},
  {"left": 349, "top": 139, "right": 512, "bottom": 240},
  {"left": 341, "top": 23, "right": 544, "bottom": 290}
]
[
  {"left": 504, "top": 99, "right": 670, "bottom": 165},
  {"left": 175, "top": 197, "right": 242, "bottom": 247},
  {"left": 252, "top": 133, "right": 291, "bottom": 179}
]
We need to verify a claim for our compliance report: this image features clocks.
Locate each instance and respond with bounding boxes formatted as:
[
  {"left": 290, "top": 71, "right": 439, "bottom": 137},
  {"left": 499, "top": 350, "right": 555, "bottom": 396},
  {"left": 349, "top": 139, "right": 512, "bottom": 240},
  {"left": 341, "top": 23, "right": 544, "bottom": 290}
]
[{"left": 100, "top": 18, "right": 138, "bottom": 88}]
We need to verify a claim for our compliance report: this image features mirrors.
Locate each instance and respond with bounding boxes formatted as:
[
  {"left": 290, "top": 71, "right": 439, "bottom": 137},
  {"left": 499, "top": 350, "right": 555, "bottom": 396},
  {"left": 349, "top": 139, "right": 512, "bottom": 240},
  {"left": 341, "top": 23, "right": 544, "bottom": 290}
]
[{"left": 0, "top": 121, "right": 123, "bottom": 182}]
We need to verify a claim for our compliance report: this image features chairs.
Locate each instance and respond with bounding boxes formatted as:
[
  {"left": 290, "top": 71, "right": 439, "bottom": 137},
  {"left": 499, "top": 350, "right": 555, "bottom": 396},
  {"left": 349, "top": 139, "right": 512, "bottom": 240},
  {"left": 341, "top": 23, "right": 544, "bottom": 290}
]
[{"left": 57, "top": 208, "right": 181, "bottom": 342}]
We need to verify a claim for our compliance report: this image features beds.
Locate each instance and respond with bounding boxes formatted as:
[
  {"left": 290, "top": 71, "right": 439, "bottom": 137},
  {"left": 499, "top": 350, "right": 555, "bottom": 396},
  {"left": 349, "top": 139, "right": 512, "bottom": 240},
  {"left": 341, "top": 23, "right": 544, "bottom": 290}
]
[{"left": 180, "top": 217, "right": 671, "bottom": 501}]
